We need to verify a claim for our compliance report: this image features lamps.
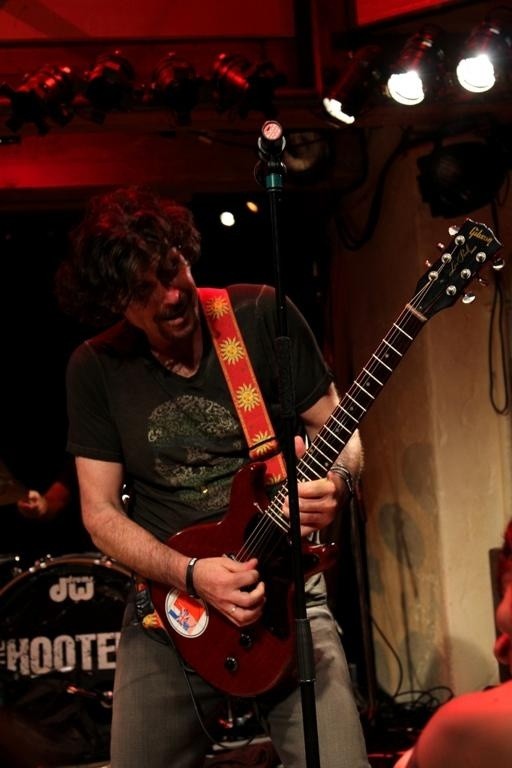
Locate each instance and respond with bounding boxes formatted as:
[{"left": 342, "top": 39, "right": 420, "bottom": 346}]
[
  {"left": 3, "top": 43, "right": 280, "bottom": 149},
  {"left": 320, "top": 18, "right": 512, "bottom": 128}
]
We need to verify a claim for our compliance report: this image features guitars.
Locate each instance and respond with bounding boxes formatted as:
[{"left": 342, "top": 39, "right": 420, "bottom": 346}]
[{"left": 143, "top": 216, "right": 506, "bottom": 699}]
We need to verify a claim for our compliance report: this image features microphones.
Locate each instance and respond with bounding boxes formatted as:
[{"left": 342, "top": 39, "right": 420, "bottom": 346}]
[{"left": 254, "top": 122, "right": 287, "bottom": 189}]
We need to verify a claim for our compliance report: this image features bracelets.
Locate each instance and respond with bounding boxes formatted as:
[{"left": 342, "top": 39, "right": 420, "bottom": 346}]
[
  {"left": 330, "top": 464, "right": 355, "bottom": 499},
  {"left": 186, "top": 556, "right": 201, "bottom": 598}
]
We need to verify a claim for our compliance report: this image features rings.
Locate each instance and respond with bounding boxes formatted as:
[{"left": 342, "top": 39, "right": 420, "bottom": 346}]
[{"left": 228, "top": 605, "right": 238, "bottom": 613}]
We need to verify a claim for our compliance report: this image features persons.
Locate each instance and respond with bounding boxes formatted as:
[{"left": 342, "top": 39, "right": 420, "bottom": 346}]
[
  {"left": 60, "top": 186, "right": 369, "bottom": 767},
  {"left": 17, "top": 482, "right": 69, "bottom": 517},
  {"left": 409, "top": 520, "right": 512, "bottom": 767}
]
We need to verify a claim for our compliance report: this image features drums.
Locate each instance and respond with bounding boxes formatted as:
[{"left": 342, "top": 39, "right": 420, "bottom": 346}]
[{"left": 0, "top": 536, "right": 139, "bottom": 762}]
